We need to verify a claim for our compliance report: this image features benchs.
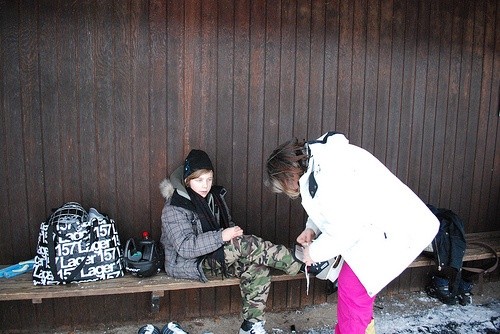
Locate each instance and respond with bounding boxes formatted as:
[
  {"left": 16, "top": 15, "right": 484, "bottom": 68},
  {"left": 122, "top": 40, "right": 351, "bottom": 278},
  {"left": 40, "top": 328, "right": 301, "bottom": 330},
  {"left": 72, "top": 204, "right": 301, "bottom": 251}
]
[{"left": 0, "top": 231, "right": 500, "bottom": 313}]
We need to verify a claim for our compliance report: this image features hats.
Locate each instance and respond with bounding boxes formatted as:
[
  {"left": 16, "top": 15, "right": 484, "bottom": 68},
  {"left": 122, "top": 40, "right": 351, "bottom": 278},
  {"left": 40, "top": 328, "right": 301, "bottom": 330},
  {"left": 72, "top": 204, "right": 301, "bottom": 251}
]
[{"left": 183, "top": 149, "right": 214, "bottom": 180}]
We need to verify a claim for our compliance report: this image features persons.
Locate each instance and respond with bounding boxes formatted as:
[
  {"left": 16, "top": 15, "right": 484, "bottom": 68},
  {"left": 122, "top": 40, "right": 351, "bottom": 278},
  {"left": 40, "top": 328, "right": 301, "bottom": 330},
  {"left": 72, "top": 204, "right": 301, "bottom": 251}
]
[
  {"left": 160, "top": 149, "right": 345, "bottom": 334},
  {"left": 264, "top": 130, "right": 441, "bottom": 334}
]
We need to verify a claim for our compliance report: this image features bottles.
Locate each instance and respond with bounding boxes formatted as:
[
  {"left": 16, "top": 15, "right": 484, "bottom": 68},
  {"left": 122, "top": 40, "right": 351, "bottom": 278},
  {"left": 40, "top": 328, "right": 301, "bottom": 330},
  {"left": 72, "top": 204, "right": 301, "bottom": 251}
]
[{"left": 142, "top": 231, "right": 152, "bottom": 241}]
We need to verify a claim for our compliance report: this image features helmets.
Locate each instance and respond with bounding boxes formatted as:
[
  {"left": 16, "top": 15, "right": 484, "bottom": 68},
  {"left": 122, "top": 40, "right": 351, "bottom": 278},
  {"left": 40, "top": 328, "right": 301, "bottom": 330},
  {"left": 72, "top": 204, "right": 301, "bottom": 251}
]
[{"left": 122, "top": 237, "right": 164, "bottom": 280}]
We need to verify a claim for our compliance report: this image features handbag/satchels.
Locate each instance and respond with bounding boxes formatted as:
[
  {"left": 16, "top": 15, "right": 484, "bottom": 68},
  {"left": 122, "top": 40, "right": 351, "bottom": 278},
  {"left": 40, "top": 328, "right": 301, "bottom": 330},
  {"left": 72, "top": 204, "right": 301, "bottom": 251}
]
[
  {"left": 31, "top": 202, "right": 127, "bottom": 286},
  {"left": 423, "top": 204, "right": 465, "bottom": 259}
]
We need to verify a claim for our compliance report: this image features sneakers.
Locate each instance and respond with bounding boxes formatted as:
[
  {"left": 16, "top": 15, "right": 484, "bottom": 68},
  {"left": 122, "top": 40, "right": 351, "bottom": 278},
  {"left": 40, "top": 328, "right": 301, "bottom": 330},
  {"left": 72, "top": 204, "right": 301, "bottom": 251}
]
[
  {"left": 138, "top": 322, "right": 189, "bottom": 334},
  {"left": 427, "top": 284, "right": 456, "bottom": 305},
  {"left": 457, "top": 293, "right": 472, "bottom": 306},
  {"left": 292, "top": 243, "right": 345, "bottom": 282},
  {"left": 239, "top": 319, "right": 268, "bottom": 334}
]
